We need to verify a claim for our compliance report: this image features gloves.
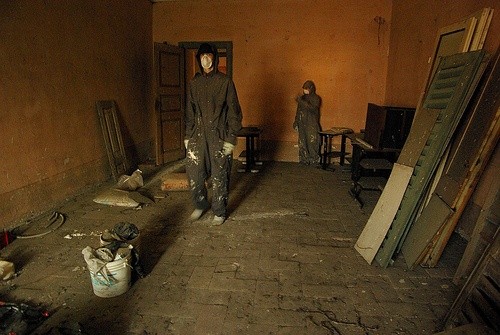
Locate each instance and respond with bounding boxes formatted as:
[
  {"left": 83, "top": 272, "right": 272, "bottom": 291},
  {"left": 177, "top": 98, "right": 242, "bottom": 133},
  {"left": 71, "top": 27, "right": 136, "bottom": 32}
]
[
  {"left": 223, "top": 141, "right": 234, "bottom": 155},
  {"left": 184, "top": 139, "right": 189, "bottom": 149},
  {"left": 293, "top": 122, "right": 298, "bottom": 129}
]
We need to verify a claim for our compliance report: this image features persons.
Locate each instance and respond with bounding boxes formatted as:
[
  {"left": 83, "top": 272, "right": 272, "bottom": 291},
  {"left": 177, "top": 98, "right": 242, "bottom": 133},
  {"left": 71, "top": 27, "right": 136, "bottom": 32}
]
[
  {"left": 183, "top": 42, "right": 243, "bottom": 225},
  {"left": 293, "top": 80, "right": 322, "bottom": 167}
]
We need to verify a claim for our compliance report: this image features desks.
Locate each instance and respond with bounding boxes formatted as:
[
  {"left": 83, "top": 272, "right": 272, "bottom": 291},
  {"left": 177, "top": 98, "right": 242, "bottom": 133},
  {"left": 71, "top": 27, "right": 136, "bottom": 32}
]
[
  {"left": 235, "top": 127, "right": 264, "bottom": 173},
  {"left": 318, "top": 129, "right": 402, "bottom": 208}
]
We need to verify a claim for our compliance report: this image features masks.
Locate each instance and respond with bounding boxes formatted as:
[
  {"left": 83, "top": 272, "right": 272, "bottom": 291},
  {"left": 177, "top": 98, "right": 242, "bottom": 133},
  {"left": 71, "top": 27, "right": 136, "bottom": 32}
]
[
  {"left": 200, "top": 55, "right": 213, "bottom": 69},
  {"left": 303, "top": 89, "right": 310, "bottom": 95}
]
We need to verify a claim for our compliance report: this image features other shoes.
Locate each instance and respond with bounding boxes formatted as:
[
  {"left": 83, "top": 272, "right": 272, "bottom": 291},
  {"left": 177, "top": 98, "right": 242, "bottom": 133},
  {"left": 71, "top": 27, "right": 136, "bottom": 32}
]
[
  {"left": 298, "top": 161, "right": 305, "bottom": 168},
  {"left": 311, "top": 163, "right": 320, "bottom": 169},
  {"left": 212, "top": 214, "right": 226, "bottom": 226},
  {"left": 190, "top": 209, "right": 204, "bottom": 220}
]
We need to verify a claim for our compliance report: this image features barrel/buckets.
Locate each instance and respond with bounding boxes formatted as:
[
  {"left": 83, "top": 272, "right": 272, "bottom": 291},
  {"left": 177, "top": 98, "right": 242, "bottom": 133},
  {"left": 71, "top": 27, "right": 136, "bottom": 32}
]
[
  {"left": 99, "top": 231, "right": 140, "bottom": 273},
  {"left": 84, "top": 246, "right": 132, "bottom": 297}
]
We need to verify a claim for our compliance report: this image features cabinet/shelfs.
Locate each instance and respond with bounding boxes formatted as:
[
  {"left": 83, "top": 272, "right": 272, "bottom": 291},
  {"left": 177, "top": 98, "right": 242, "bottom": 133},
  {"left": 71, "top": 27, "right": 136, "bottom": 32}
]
[{"left": 363, "top": 102, "right": 416, "bottom": 151}]
